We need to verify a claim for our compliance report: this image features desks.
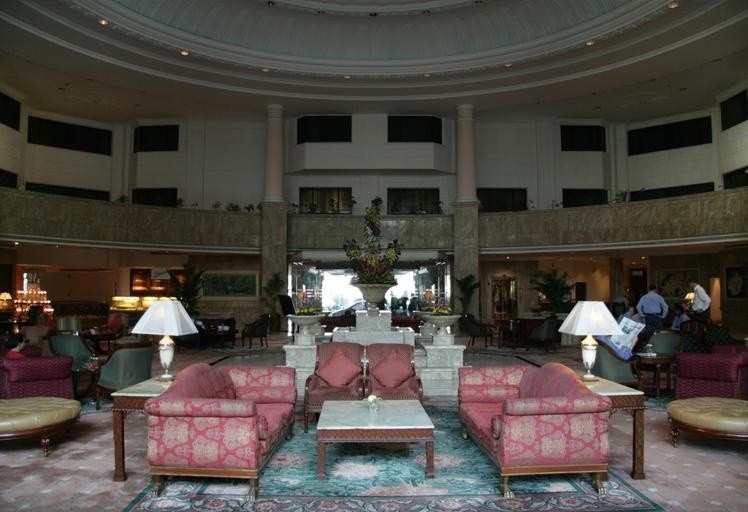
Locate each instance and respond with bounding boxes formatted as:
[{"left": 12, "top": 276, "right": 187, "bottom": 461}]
[
  {"left": 496, "top": 319, "right": 530, "bottom": 351},
  {"left": 638, "top": 355, "right": 673, "bottom": 400},
  {"left": 109, "top": 375, "right": 175, "bottom": 482},
  {"left": 575, "top": 369, "right": 649, "bottom": 481}
]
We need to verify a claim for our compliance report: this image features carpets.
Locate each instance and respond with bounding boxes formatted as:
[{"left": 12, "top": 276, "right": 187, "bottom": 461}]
[
  {"left": 76, "top": 393, "right": 115, "bottom": 417},
  {"left": 116, "top": 403, "right": 666, "bottom": 512}
]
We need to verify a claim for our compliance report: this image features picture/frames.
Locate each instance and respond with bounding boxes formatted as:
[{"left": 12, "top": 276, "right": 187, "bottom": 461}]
[
  {"left": 654, "top": 267, "right": 701, "bottom": 303},
  {"left": 198, "top": 269, "right": 262, "bottom": 303},
  {"left": 722, "top": 263, "right": 748, "bottom": 301}
]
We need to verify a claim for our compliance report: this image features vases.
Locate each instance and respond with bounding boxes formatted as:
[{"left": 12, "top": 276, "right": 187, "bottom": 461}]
[
  {"left": 352, "top": 283, "right": 396, "bottom": 332},
  {"left": 412, "top": 310, "right": 433, "bottom": 329},
  {"left": 286, "top": 314, "right": 327, "bottom": 336},
  {"left": 421, "top": 314, "right": 463, "bottom": 336}
]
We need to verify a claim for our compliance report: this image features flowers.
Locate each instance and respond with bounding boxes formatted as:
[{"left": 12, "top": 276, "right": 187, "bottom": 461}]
[
  {"left": 293, "top": 305, "right": 324, "bottom": 316},
  {"left": 430, "top": 305, "right": 454, "bottom": 316},
  {"left": 342, "top": 195, "right": 405, "bottom": 284}
]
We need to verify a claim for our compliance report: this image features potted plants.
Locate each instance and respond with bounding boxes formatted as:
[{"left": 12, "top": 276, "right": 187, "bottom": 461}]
[{"left": 450, "top": 274, "right": 481, "bottom": 333}]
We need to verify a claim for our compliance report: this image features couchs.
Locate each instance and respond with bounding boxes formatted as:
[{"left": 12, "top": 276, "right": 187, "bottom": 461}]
[
  {"left": 463, "top": 320, "right": 496, "bottom": 349},
  {"left": 526, "top": 318, "right": 563, "bottom": 354},
  {"left": 0, "top": 345, "right": 74, "bottom": 399},
  {"left": 679, "top": 319, "right": 708, "bottom": 340},
  {"left": 41, "top": 334, "right": 96, "bottom": 400},
  {"left": 144, "top": 361, "right": 299, "bottom": 505},
  {"left": 665, "top": 396, "right": 748, "bottom": 448},
  {"left": 645, "top": 332, "right": 681, "bottom": 389},
  {"left": 106, "top": 311, "right": 131, "bottom": 337},
  {"left": 674, "top": 343, "right": 748, "bottom": 400},
  {"left": 241, "top": 312, "right": 272, "bottom": 349},
  {"left": 303, "top": 341, "right": 365, "bottom": 434},
  {"left": 95, "top": 343, "right": 155, "bottom": 411},
  {"left": 580, "top": 337, "right": 643, "bottom": 389},
  {"left": 364, "top": 343, "right": 423, "bottom": 406},
  {"left": 0, "top": 396, "right": 82, "bottom": 458},
  {"left": 456, "top": 360, "right": 613, "bottom": 499},
  {"left": 52, "top": 314, "right": 101, "bottom": 347},
  {"left": 128, "top": 266, "right": 188, "bottom": 297},
  {"left": 195, "top": 316, "right": 239, "bottom": 349},
  {"left": 611, "top": 301, "right": 626, "bottom": 322}
]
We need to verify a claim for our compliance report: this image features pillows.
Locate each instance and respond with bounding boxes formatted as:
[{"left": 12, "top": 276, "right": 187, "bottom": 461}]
[
  {"left": 705, "top": 322, "right": 730, "bottom": 345},
  {"left": 680, "top": 334, "right": 713, "bottom": 353}
]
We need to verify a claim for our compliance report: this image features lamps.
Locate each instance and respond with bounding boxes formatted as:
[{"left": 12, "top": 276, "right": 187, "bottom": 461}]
[
  {"left": 666, "top": 1, "right": 679, "bottom": 10},
  {"left": 0, "top": 291, "right": 13, "bottom": 322},
  {"left": 683, "top": 292, "right": 696, "bottom": 315},
  {"left": 130, "top": 300, "right": 200, "bottom": 383},
  {"left": 558, "top": 300, "right": 625, "bottom": 382},
  {"left": 12, "top": 282, "right": 55, "bottom": 312},
  {"left": 179, "top": 49, "right": 190, "bottom": 57},
  {"left": 98, "top": 18, "right": 110, "bottom": 27}
]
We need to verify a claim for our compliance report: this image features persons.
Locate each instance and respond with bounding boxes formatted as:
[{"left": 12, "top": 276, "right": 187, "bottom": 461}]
[
  {"left": 670, "top": 304, "right": 690, "bottom": 332},
  {"left": 617, "top": 305, "right": 636, "bottom": 324},
  {"left": 688, "top": 277, "right": 712, "bottom": 326},
  {"left": 594, "top": 334, "right": 642, "bottom": 366},
  {"left": 4, "top": 334, "right": 26, "bottom": 360},
  {"left": 635, "top": 283, "right": 668, "bottom": 330}
]
